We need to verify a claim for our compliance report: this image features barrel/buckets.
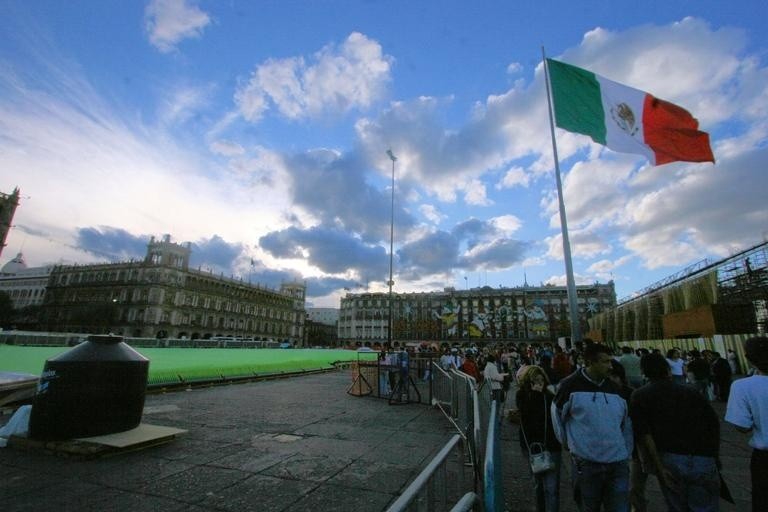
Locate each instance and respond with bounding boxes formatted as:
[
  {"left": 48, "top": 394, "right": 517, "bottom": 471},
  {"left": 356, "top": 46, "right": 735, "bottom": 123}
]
[{"left": 29, "top": 336, "right": 149, "bottom": 439}]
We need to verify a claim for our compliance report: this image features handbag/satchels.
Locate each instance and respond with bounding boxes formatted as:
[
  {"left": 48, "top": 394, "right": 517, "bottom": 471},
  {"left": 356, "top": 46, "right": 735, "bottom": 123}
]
[{"left": 529, "top": 450, "right": 555, "bottom": 475}]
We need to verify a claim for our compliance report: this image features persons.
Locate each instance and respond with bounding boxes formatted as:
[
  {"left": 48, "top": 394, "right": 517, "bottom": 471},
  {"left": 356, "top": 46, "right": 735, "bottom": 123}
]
[
  {"left": 379, "top": 341, "right": 409, "bottom": 397},
  {"left": 610, "top": 345, "right": 736, "bottom": 417},
  {"left": 515, "top": 365, "right": 562, "bottom": 512},
  {"left": 724, "top": 336, "right": 768, "bottom": 512},
  {"left": 551, "top": 343, "right": 634, "bottom": 512},
  {"left": 407, "top": 342, "right": 439, "bottom": 380},
  {"left": 438, "top": 342, "right": 585, "bottom": 415},
  {"left": 629, "top": 353, "right": 722, "bottom": 512}
]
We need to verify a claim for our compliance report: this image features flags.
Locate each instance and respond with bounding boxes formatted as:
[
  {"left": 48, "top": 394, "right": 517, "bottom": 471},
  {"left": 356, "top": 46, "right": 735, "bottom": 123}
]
[{"left": 545, "top": 56, "right": 716, "bottom": 165}]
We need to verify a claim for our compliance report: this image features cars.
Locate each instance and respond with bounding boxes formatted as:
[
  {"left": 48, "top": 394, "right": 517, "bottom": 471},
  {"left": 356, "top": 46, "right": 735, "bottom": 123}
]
[{"left": 279, "top": 343, "right": 292, "bottom": 349}]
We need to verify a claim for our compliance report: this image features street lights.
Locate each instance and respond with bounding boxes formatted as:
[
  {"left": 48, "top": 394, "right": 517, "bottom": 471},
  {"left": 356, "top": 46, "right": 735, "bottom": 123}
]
[{"left": 385, "top": 148, "right": 397, "bottom": 346}]
